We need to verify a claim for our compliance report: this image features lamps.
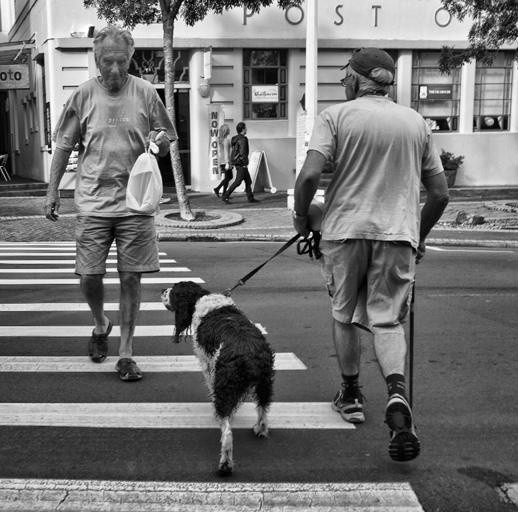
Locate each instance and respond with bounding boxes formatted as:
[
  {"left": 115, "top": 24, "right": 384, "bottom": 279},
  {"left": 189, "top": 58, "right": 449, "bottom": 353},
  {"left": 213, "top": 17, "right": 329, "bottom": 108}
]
[{"left": 199, "top": 76, "right": 210, "bottom": 98}]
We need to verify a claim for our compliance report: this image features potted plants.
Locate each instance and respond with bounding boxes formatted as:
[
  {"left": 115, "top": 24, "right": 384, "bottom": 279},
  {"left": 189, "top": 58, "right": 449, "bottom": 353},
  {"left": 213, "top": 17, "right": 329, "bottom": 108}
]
[{"left": 439, "top": 147, "right": 466, "bottom": 188}]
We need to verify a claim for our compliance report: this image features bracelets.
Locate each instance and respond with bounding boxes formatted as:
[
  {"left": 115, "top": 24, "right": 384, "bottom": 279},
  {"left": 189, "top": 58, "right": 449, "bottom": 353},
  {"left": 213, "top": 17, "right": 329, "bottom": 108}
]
[{"left": 293, "top": 210, "right": 306, "bottom": 221}]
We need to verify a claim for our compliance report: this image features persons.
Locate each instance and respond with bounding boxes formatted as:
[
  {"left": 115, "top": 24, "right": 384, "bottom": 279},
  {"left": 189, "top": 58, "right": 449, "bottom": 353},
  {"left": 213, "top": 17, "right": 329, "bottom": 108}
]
[
  {"left": 295, "top": 48, "right": 449, "bottom": 462},
  {"left": 221, "top": 122, "right": 260, "bottom": 202},
  {"left": 213, "top": 124, "right": 231, "bottom": 198},
  {"left": 44, "top": 25, "right": 178, "bottom": 381}
]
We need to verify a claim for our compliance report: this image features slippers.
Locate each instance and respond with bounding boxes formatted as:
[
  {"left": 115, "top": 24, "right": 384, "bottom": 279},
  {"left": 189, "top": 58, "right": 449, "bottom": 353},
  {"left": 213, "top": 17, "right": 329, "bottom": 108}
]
[
  {"left": 114, "top": 356, "right": 144, "bottom": 381},
  {"left": 87, "top": 314, "right": 113, "bottom": 362}
]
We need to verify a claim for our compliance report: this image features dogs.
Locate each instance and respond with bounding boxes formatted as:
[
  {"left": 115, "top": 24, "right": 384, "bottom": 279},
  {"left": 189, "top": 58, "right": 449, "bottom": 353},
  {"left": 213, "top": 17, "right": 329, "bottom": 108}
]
[{"left": 160, "top": 281, "right": 275, "bottom": 476}]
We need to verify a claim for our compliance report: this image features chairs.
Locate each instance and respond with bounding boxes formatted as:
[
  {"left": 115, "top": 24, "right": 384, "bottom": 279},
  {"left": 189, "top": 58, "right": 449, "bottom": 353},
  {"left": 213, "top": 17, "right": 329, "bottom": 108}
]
[{"left": 0, "top": 153, "right": 14, "bottom": 185}]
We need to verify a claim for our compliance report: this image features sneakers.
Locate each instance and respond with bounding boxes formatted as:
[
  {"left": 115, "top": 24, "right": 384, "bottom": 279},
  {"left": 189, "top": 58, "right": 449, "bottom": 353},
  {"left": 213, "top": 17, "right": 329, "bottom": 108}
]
[
  {"left": 248, "top": 198, "right": 260, "bottom": 203},
  {"left": 222, "top": 196, "right": 231, "bottom": 204},
  {"left": 213, "top": 188, "right": 220, "bottom": 197},
  {"left": 332, "top": 387, "right": 366, "bottom": 422},
  {"left": 384, "top": 394, "right": 421, "bottom": 462}
]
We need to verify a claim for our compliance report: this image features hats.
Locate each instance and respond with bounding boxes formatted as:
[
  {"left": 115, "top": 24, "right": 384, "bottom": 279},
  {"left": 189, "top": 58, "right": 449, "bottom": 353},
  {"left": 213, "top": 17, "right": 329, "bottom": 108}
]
[{"left": 340, "top": 45, "right": 395, "bottom": 85}]
